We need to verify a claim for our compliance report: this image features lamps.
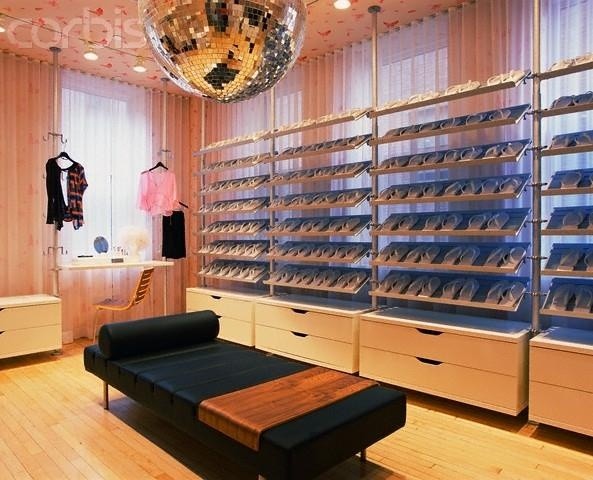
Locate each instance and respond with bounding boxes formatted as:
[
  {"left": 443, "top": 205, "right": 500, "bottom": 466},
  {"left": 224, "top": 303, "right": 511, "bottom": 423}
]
[{"left": 132, "top": 0, "right": 313, "bottom": 106}]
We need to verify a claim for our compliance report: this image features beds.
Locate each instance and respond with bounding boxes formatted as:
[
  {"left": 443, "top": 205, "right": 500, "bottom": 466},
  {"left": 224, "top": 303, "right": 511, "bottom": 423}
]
[{"left": 80, "top": 307, "right": 409, "bottom": 477}]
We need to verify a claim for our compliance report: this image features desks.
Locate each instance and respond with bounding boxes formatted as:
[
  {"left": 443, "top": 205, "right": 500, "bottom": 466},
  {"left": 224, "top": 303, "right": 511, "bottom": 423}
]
[{"left": 51, "top": 261, "right": 174, "bottom": 348}]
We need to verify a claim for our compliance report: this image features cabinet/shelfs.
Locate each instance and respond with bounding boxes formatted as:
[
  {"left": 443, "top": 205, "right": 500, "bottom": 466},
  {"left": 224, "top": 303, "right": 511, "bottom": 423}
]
[
  {"left": 0, "top": 292, "right": 62, "bottom": 362},
  {"left": 523, "top": 322, "right": 592, "bottom": 439},
  {"left": 254, "top": 290, "right": 394, "bottom": 374},
  {"left": 359, "top": 304, "right": 533, "bottom": 417},
  {"left": 184, "top": 279, "right": 289, "bottom": 349},
  {"left": 183, "top": 59, "right": 593, "bottom": 341}
]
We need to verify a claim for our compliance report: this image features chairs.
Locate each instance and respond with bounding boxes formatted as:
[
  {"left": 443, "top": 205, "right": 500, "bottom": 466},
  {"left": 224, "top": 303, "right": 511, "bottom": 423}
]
[{"left": 83, "top": 262, "right": 154, "bottom": 345}]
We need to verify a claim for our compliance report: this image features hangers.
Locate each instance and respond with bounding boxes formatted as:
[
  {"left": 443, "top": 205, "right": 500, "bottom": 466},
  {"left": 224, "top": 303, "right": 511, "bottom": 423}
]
[
  {"left": 46, "top": 138, "right": 78, "bottom": 171},
  {"left": 137, "top": 149, "right": 171, "bottom": 175},
  {"left": 175, "top": 198, "right": 189, "bottom": 210}
]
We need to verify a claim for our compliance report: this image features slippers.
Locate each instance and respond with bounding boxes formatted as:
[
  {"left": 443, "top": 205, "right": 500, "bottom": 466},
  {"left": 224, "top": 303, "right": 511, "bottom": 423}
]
[
  {"left": 269, "top": 108, "right": 370, "bottom": 293},
  {"left": 550, "top": 55, "right": 593, "bottom": 318},
  {"left": 375, "top": 71, "right": 519, "bottom": 307},
  {"left": 197, "top": 130, "right": 265, "bottom": 281}
]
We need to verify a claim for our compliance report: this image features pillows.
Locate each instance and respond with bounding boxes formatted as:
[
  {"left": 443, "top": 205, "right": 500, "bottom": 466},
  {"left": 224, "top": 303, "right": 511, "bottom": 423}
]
[{"left": 97, "top": 304, "right": 221, "bottom": 356}]
